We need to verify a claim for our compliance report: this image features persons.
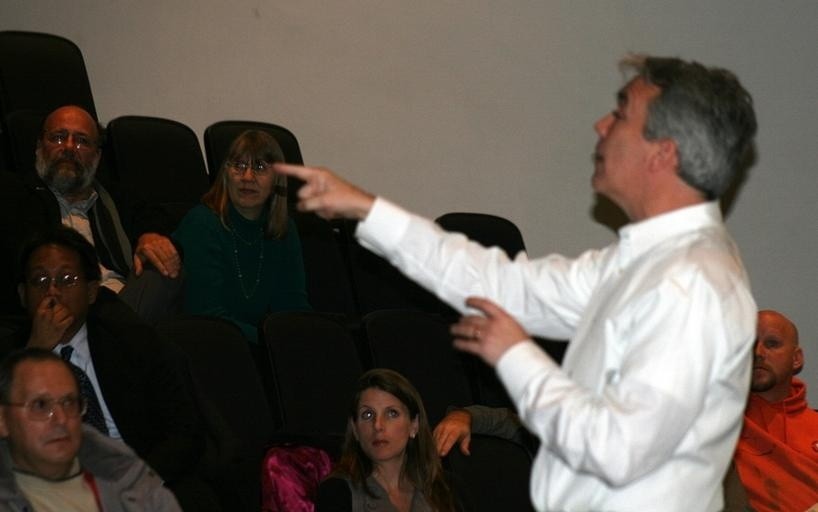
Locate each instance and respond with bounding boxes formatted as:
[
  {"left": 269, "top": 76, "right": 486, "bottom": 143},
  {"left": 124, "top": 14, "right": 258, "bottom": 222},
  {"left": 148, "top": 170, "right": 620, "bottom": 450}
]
[
  {"left": 1, "top": 225, "right": 191, "bottom": 497},
  {"left": 734, "top": 311, "right": 817, "bottom": 512},
  {"left": 178, "top": 129, "right": 309, "bottom": 437},
  {"left": 273, "top": 56, "right": 757, "bottom": 512},
  {"left": 0, "top": 349, "right": 180, "bottom": 511},
  {"left": 0, "top": 105, "right": 179, "bottom": 325},
  {"left": 315, "top": 369, "right": 456, "bottom": 511},
  {"left": 432, "top": 404, "right": 755, "bottom": 511}
]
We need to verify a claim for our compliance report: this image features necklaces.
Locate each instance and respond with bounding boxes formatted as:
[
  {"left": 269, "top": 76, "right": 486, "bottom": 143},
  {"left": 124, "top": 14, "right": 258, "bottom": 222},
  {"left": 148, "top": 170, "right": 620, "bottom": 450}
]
[{"left": 226, "top": 217, "right": 265, "bottom": 299}]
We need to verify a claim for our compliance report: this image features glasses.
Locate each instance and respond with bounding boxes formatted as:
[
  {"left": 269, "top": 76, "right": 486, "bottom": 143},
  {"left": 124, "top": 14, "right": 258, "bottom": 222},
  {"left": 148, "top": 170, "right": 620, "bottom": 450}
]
[
  {"left": 41, "top": 133, "right": 97, "bottom": 150},
  {"left": 3, "top": 395, "right": 88, "bottom": 422},
  {"left": 23, "top": 271, "right": 88, "bottom": 289},
  {"left": 227, "top": 161, "right": 272, "bottom": 173}
]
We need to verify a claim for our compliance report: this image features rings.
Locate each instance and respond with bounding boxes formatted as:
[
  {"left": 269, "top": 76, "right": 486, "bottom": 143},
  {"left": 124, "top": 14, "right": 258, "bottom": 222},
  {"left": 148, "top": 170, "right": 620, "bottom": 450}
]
[{"left": 472, "top": 324, "right": 480, "bottom": 339}]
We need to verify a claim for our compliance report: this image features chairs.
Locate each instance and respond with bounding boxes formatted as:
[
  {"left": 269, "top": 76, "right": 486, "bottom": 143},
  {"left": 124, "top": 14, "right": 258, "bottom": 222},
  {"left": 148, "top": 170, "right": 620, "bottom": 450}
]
[
  {"left": 433, "top": 213, "right": 526, "bottom": 260},
  {"left": 0, "top": 30, "right": 99, "bottom": 156},
  {"left": 106, "top": 115, "right": 207, "bottom": 225},
  {"left": 204, "top": 120, "right": 303, "bottom": 190},
  {"left": 263, "top": 448, "right": 331, "bottom": 512}
]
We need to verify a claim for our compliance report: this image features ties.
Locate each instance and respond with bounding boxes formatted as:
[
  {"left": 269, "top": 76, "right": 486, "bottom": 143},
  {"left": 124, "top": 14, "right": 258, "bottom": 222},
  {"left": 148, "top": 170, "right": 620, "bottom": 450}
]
[{"left": 60, "top": 346, "right": 109, "bottom": 436}]
